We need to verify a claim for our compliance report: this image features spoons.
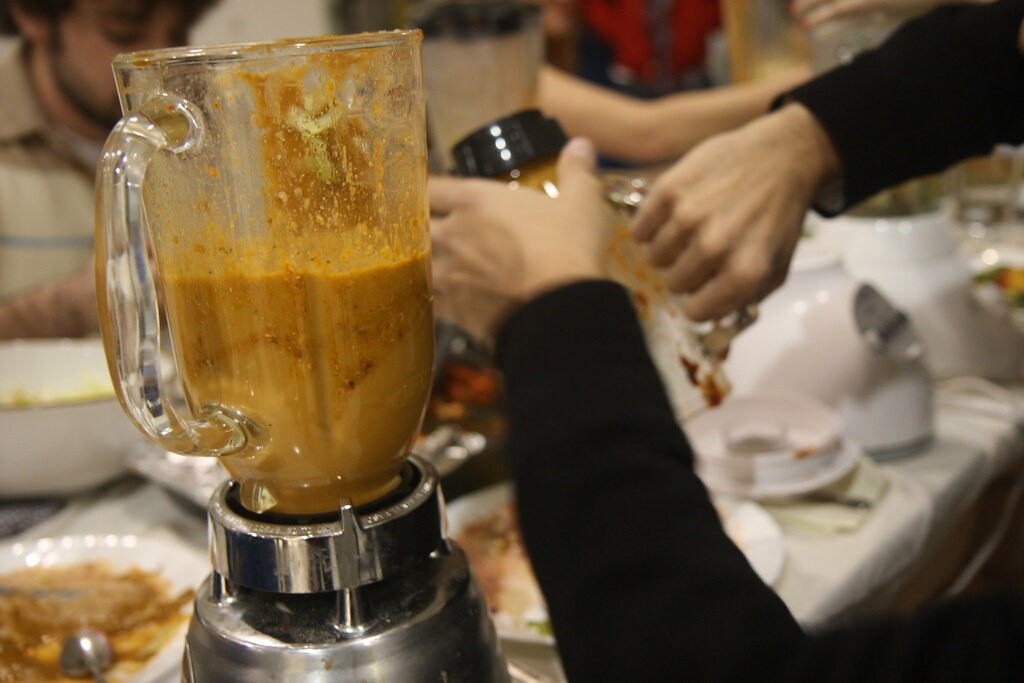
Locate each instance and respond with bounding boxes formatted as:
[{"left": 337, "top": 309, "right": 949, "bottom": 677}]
[{"left": 60, "top": 629, "right": 109, "bottom": 683}]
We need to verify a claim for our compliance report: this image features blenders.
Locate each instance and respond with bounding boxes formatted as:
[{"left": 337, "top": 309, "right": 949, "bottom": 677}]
[{"left": 96, "top": 28, "right": 511, "bottom": 683}]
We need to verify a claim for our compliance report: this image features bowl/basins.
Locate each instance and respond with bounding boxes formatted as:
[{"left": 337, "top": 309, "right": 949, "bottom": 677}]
[
  {"left": 691, "top": 386, "right": 844, "bottom": 484},
  {"left": 0, "top": 339, "right": 175, "bottom": 498}
]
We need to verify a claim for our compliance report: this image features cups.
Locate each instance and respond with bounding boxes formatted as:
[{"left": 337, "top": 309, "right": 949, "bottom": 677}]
[{"left": 428, "top": 109, "right": 732, "bottom": 423}]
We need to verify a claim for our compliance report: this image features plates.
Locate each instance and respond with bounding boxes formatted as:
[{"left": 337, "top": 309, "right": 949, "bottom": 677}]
[
  {"left": 0, "top": 533, "right": 213, "bottom": 683},
  {"left": 444, "top": 478, "right": 786, "bottom": 646},
  {"left": 690, "top": 439, "right": 861, "bottom": 500}
]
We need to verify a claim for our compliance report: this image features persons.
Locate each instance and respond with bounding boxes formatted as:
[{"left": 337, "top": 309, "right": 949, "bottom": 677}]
[
  {"left": 426, "top": 2, "right": 1024, "bottom": 682},
  {"left": 0, "top": 1, "right": 227, "bottom": 342}
]
